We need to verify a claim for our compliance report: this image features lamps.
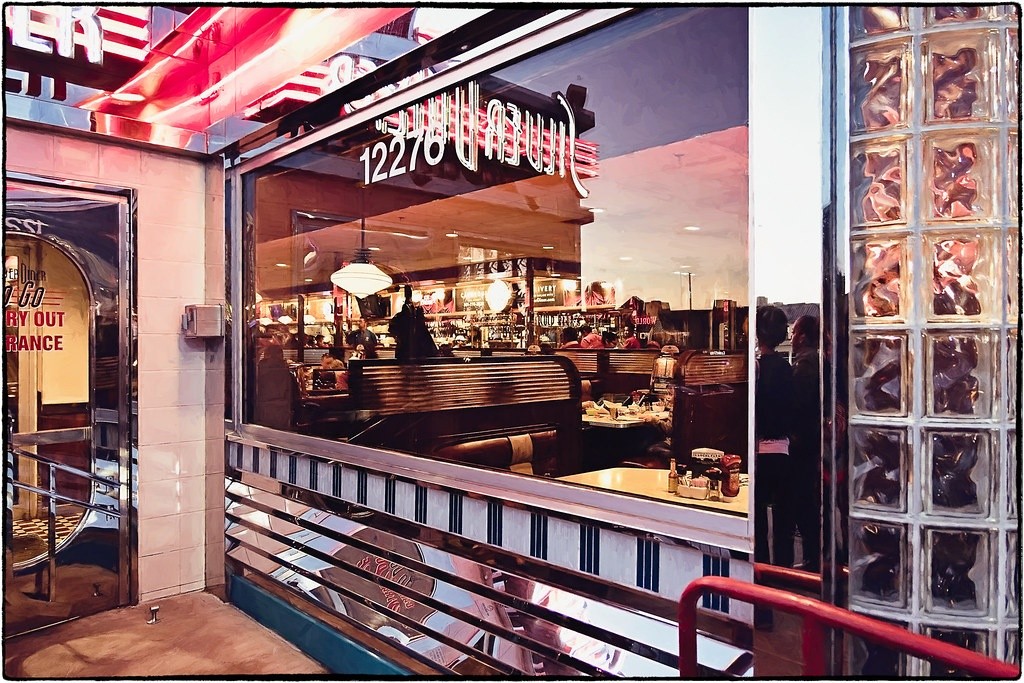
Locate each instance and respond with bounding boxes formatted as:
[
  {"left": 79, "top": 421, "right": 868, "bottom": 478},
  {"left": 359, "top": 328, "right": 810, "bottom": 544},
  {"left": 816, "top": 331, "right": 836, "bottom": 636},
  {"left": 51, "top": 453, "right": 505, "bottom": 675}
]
[
  {"left": 329, "top": 180, "right": 393, "bottom": 298},
  {"left": 256, "top": 293, "right": 317, "bottom": 327}
]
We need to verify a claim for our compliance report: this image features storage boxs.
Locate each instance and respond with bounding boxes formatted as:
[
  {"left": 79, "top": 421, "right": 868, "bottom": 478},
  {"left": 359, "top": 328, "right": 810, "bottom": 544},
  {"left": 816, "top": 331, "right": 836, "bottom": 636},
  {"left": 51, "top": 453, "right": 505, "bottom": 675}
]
[{"left": 185, "top": 303, "right": 226, "bottom": 339}]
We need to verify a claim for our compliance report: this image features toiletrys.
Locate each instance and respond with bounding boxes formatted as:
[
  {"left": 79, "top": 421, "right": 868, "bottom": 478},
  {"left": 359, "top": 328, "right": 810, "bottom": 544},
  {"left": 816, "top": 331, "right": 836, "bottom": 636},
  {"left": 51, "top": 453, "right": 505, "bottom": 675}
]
[{"left": 668, "top": 449, "right": 742, "bottom": 502}]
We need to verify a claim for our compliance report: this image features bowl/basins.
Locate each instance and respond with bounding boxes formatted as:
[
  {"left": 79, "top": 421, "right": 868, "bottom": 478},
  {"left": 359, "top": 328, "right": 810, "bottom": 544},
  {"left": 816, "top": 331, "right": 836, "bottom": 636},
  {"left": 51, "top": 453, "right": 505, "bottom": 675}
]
[
  {"left": 652, "top": 405, "right": 665, "bottom": 412},
  {"left": 586, "top": 409, "right": 599, "bottom": 416}
]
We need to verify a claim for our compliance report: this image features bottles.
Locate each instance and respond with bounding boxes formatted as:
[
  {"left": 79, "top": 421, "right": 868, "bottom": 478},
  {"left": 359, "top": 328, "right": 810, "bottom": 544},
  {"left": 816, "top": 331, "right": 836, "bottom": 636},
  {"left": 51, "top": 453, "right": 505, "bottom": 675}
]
[{"left": 668, "top": 458, "right": 679, "bottom": 492}]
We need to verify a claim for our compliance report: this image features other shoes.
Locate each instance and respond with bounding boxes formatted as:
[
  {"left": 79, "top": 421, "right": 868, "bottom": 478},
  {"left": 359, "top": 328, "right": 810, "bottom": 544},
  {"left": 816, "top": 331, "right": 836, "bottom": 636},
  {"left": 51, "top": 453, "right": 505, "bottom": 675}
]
[
  {"left": 754, "top": 621, "right": 774, "bottom": 633},
  {"left": 791, "top": 562, "right": 817, "bottom": 571}
]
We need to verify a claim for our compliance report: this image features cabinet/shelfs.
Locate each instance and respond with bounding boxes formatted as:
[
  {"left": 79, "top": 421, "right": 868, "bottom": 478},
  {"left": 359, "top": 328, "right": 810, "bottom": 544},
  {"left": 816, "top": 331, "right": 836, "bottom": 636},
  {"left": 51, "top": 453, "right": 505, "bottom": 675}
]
[{"left": 383, "top": 305, "right": 615, "bottom": 345}]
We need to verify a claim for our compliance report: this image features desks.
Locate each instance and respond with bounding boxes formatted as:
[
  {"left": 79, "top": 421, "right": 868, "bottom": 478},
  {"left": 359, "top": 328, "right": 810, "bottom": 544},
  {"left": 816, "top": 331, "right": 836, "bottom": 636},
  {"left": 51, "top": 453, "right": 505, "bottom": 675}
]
[{"left": 555, "top": 467, "right": 748, "bottom": 518}]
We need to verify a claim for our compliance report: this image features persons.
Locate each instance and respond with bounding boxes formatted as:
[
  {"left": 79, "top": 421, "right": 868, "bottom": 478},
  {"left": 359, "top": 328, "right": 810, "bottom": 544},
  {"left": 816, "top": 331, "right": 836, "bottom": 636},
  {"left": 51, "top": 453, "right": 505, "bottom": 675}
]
[
  {"left": 345, "top": 315, "right": 377, "bottom": 359},
  {"left": 264, "top": 315, "right": 661, "bottom": 390},
  {"left": 755, "top": 304, "right": 820, "bottom": 574}
]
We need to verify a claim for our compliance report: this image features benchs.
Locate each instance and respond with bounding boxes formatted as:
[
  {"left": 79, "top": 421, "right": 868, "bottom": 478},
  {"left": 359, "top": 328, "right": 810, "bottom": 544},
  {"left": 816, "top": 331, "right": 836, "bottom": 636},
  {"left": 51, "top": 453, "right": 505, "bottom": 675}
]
[
  {"left": 299, "top": 389, "right": 349, "bottom": 439},
  {"left": 644, "top": 382, "right": 737, "bottom": 474},
  {"left": 432, "top": 422, "right": 564, "bottom": 481}
]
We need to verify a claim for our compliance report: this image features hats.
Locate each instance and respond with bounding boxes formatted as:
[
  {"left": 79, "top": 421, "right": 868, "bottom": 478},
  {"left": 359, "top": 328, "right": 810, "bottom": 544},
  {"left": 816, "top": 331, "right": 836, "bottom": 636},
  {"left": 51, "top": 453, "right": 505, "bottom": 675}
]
[
  {"left": 314, "top": 335, "right": 325, "bottom": 338},
  {"left": 576, "top": 322, "right": 590, "bottom": 331},
  {"left": 453, "top": 335, "right": 468, "bottom": 341}
]
